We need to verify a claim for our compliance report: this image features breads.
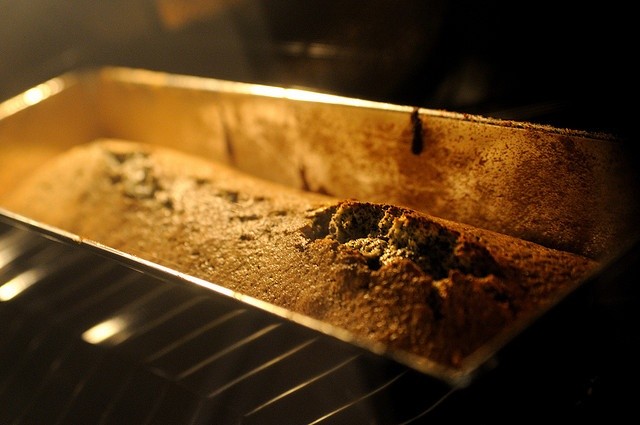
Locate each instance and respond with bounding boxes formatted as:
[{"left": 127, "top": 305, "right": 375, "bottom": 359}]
[{"left": 3, "top": 135, "right": 605, "bottom": 368}]
